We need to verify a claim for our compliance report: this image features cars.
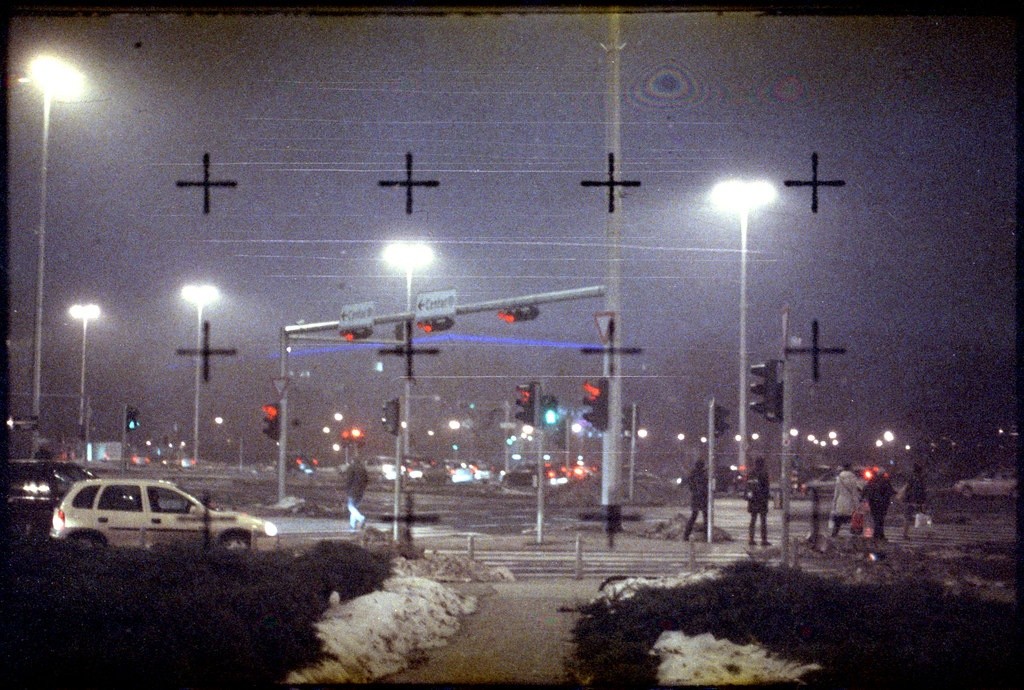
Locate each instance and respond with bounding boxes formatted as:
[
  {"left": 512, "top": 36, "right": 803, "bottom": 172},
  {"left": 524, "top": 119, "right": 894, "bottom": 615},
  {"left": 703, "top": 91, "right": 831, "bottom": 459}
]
[
  {"left": 7, "top": 461, "right": 101, "bottom": 509},
  {"left": 362, "top": 454, "right": 496, "bottom": 485},
  {"left": 499, "top": 462, "right": 602, "bottom": 490},
  {"left": 49, "top": 477, "right": 280, "bottom": 556},
  {"left": 801, "top": 465, "right": 870, "bottom": 498},
  {"left": 951, "top": 465, "right": 1017, "bottom": 502}
]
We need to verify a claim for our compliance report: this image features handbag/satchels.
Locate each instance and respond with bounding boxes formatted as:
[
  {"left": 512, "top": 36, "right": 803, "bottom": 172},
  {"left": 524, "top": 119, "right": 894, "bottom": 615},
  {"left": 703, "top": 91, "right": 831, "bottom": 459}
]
[{"left": 850, "top": 512, "right": 864, "bottom": 534}]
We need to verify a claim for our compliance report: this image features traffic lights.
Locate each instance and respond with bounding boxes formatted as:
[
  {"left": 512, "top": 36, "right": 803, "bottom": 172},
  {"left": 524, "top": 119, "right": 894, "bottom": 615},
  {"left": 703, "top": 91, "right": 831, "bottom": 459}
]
[
  {"left": 261, "top": 402, "right": 280, "bottom": 441},
  {"left": 127, "top": 408, "right": 141, "bottom": 432},
  {"left": 382, "top": 400, "right": 400, "bottom": 436},
  {"left": 516, "top": 381, "right": 560, "bottom": 431},
  {"left": 581, "top": 375, "right": 609, "bottom": 431},
  {"left": 749, "top": 360, "right": 784, "bottom": 423},
  {"left": 417, "top": 318, "right": 453, "bottom": 332},
  {"left": 339, "top": 327, "right": 373, "bottom": 340},
  {"left": 497, "top": 305, "right": 540, "bottom": 323},
  {"left": 712, "top": 406, "right": 731, "bottom": 434}
]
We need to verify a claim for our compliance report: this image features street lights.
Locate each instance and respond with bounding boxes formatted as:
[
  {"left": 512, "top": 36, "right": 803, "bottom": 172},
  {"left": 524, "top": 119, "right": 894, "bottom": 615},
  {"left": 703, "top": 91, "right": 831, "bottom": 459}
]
[
  {"left": 182, "top": 284, "right": 217, "bottom": 463},
  {"left": 385, "top": 241, "right": 433, "bottom": 456},
  {"left": 69, "top": 304, "right": 101, "bottom": 462},
  {"left": 21, "top": 55, "right": 86, "bottom": 417},
  {"left": 705, "top": 177, "right": 776, "bottom": 471}
]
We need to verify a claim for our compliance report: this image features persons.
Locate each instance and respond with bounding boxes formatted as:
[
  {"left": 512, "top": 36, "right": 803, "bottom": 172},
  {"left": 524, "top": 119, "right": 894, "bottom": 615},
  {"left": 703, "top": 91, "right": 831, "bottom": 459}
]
[
  {"left": 831, "top": 460, "right": 934, "bottom": 542},
  {"left": 149, "top": 491, "right": 161, "bottom": 511},
  {"left": 34, "top": 445, "right": 53, "bottom": 460},
  {"left": 683, "top": 458, "right": 708, "bottom": 542},
  {"left": 744, "top": 458, "right": 772, "bottom": 546},
  {"left": 346, "top": 457, "right": 368, "bottom": 530}
]
